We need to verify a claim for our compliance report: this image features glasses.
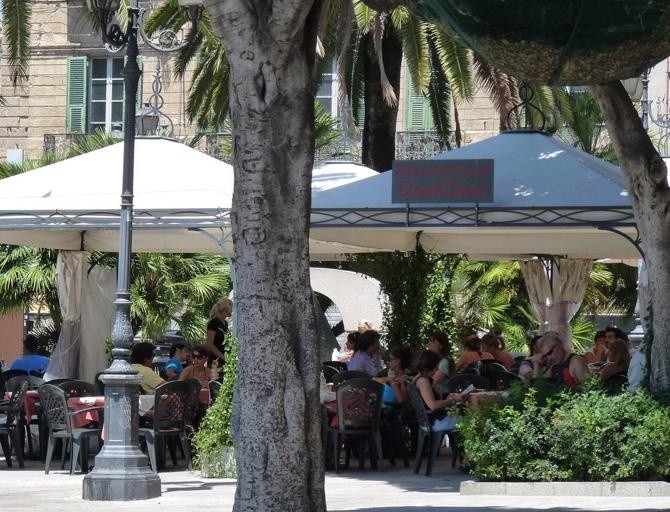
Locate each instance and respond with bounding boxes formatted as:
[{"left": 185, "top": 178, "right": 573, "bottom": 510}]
[
  {"left": 542, "top": 348, "right": 553, "bottom": 358},
  {"left": 192, "top": 353, "right": 202, "bottom": 360}
]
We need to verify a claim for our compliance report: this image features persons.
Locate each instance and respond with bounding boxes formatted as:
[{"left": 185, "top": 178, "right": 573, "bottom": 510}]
[
  {"left": 179, "top": 346, "right": 220, "bottom": 392},
  {"left": 128, "top": 342, "right": 169, "bottom": 395},
  {"left": 206, "top": 297, "right": 235, "bottom": 369},
  {"left": 10, "top": 335, "right": 50, "bottom": 374},
  {"left": 347, "top": 325, "right": 629, "bottom": 472},
  {"left": 164, "top": 343, "right": 190, "bottom": 379}
]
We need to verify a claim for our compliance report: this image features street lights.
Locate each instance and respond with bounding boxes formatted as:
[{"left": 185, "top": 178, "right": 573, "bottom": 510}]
[
  {"left": 134, "top": 103, "right": 160, "bottom": 138},
  {"left": 81, "top": 1, "right": 206, "bottom": 502}
]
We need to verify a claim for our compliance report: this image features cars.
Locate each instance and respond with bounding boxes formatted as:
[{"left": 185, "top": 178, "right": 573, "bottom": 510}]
[{"left": 133, "top": 331, "right": 186, "bottom": 357}]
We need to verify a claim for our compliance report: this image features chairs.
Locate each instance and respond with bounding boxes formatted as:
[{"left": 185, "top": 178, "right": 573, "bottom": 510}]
[
  {"left": 321, "top": 356, "right": 524, "bottom": 476},
  {"left": 0, "top": 362, "right": 223, "bottom": 475}
]
